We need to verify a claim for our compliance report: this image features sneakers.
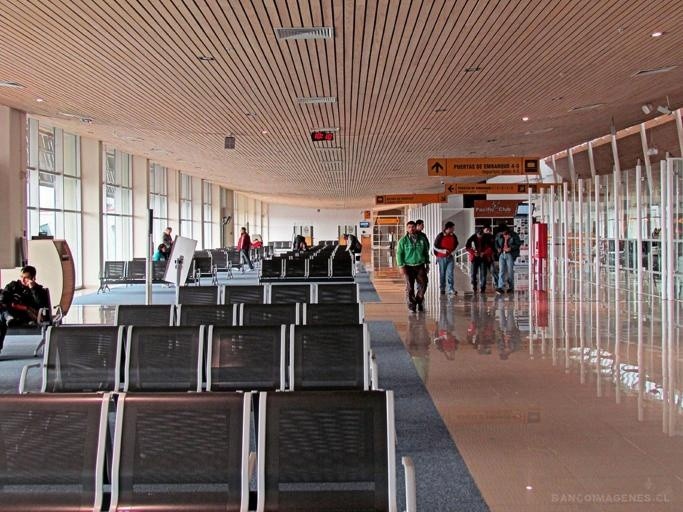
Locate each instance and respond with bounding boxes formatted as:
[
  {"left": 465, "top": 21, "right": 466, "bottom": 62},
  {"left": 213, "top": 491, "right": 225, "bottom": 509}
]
[
  {"left": 481, "top": 289, "right": 485, "bottom": 293},
  {"left": 507, "top": 289, "right": 514, "bottom": 293},
  {"left": 7, "top": 318, "right": 20, "bottom": 329},
  {"left": 473, "top": 287, "right": 477, "bottom": 292},
  {"left": 418, "top": 301, "right": 423, "bottom": 311},
  {"left": 246, "top": 269, "right": 253, "bottom": 272},
  {"left": 449, "top": 289, "right": 457, "bottom": 295},
  {"left": 496, "top": 288, "right": 505, "bottom": 294},
  {"left": 408, "top": 307, "right": 416, "bottom": 313},
  {"left": 441, "top": 290, "right": 445, "bottom": 294},
  {"left": 234, "top": 268, "right": 240, "bottom": 271}
]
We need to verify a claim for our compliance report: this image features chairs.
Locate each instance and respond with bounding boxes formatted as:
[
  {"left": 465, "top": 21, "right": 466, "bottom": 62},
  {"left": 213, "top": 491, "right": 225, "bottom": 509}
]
[
  {"left": 99, "top": 244, "right": 274, "bottom": 293},
  {"left": 116, "top": 304, "right": 175, "bottom": 325},
  {"left": 221, "top": 281, "right": 266, "bottom": 303},
  {"left": 177, "top": 303, "right": 238, "bottom": 325},
  {"left": 125, "top": 325, "right": 206, "bottom": 393},
  {"left": 302, "top": 301, "right": 363, "bottom": 324},
  {"left": 257, "top": 390, "right": 417, "bottom": 510},
  {"left": 267, "top": 282, "right": 315, "bottom": 301},
  {"left": 0, "top": 394, "right": 109, "bottom": 512},
  {"left": 174, "top": 281, "right": 221, "bottom": 306},
  {"left": 240, "top": 302, "right": 301, "bottom": 325},
  {"left": 19, "top": 322, "right": 124, "bottom": 394},
  {"left": 288, "top": 324, "right": 379, "bottom": 389},
  {"left": 258, "top": 241, "right": 355, "bottom": 282},
  {"left": 208, "top": 325, "right": 288, "bottom": 390},
  {"left": 317, "top": 282, "right": 360, "bottom": 301},
  {"left": 109, "top": 392, "right": 254, "bottom": 512}
]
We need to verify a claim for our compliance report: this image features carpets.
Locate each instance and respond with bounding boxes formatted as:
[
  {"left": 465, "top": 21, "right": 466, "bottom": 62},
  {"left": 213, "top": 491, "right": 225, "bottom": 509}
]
[
  {"left": 364, "top": 317, "right": 487, "bottom": 508},
  {"left": 359, "top": 262, "right": 381, "bottom": 306}
]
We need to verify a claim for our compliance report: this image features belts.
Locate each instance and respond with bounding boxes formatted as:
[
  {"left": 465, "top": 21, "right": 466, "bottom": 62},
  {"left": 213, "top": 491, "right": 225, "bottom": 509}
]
[{"left": 501, "top": 250, "right": 511, "bottom": 255}]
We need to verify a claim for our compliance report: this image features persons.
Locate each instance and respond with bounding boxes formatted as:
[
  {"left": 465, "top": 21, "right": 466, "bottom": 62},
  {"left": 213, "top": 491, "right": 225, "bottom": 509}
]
[
  {"left": 238, "top": 227, "right": 255, "bottom": 274},
  {"left": 495, "top": 225, "right": 520, "bottom": 295},
  {"left": 342, "top": 233, "right": 361, "bottom": 263},
  {"left": 292, "top": 235, "right": 308, "bottom": 254},
  {"left": 433, "top": 221, "right": 458, "bottom": 295},
  {"left": 0, "top": 266, "right": 52, "bottom": 329},
  {"left": 466, "top": 228, "right": 492, "bottom": 294},
  {"left": 252, "top": 238, "right": 263, "bottom": 249},
  {"left": 162, "top": 227, "right": 174, "bottom": 250},
  {"left": 152, "top": 244, "right": 168, "bottom": 262},
  {"left": 483, "top": 226, "right": 498, "bottom": 288},
  {"left": 414, "top": 220, "right": 430, "bottom": 250},
  {"left": 397, "top": 220, "right": 431, "bottom": 313}
]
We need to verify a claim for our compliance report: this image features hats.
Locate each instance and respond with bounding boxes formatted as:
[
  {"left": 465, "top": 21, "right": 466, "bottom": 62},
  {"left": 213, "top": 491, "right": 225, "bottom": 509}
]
[
  {"left": 158, "top": 244, "right": 166, "bottom": 250},
  {"left": 390, "top": 228, "right": 395, "bottom": 233}
]
